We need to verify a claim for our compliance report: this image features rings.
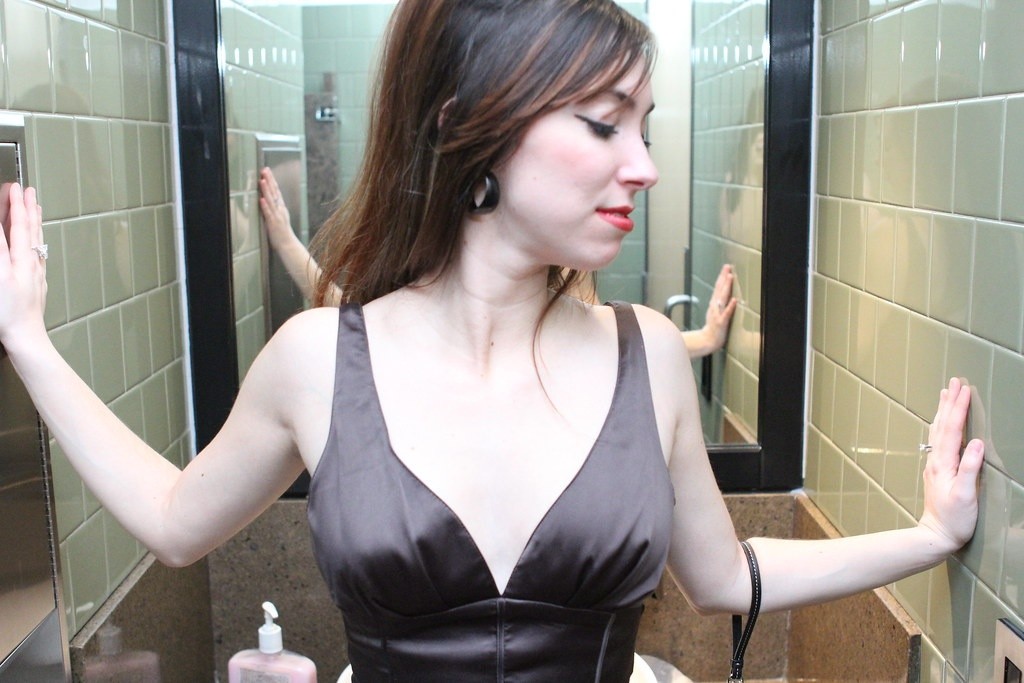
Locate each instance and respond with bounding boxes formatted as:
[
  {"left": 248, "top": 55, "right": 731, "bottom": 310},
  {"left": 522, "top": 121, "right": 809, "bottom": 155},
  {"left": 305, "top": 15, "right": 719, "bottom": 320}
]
[
  {"left": 919, "top": 444, "right": 932, "bottom": 450},
  {"left": 276, "top": 200, "right": 282, "bottom": 207},
  {"left": 717, "top": 302, "right": 725, "bottom": 307},
  {"left": 32, "top": 243, "right": 48, "bottom": 260}
]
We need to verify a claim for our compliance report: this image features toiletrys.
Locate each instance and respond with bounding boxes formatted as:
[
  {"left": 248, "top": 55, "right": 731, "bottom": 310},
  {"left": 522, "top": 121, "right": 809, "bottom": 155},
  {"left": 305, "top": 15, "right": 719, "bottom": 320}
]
[
  {"left": 82, "top": 612, "right": 162, "bottom": 683},
  {"left": 227, "top": 600, "right": 318, "bottom": 683}
]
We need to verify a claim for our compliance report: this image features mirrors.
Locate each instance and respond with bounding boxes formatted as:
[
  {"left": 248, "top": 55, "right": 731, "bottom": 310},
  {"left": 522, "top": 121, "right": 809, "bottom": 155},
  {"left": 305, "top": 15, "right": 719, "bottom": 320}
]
[{"left": 161, "top": 0, "right": 823, "bottom": 505}]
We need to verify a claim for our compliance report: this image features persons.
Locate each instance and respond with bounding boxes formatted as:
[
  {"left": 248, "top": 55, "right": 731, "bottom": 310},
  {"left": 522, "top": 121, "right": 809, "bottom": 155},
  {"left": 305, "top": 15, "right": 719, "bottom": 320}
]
[
  {"left": 259, "top": 166, "right": 736, "bottom": 360},
  {"left": 0, "top": 0, "right": 986, "bottom": 683}
]
